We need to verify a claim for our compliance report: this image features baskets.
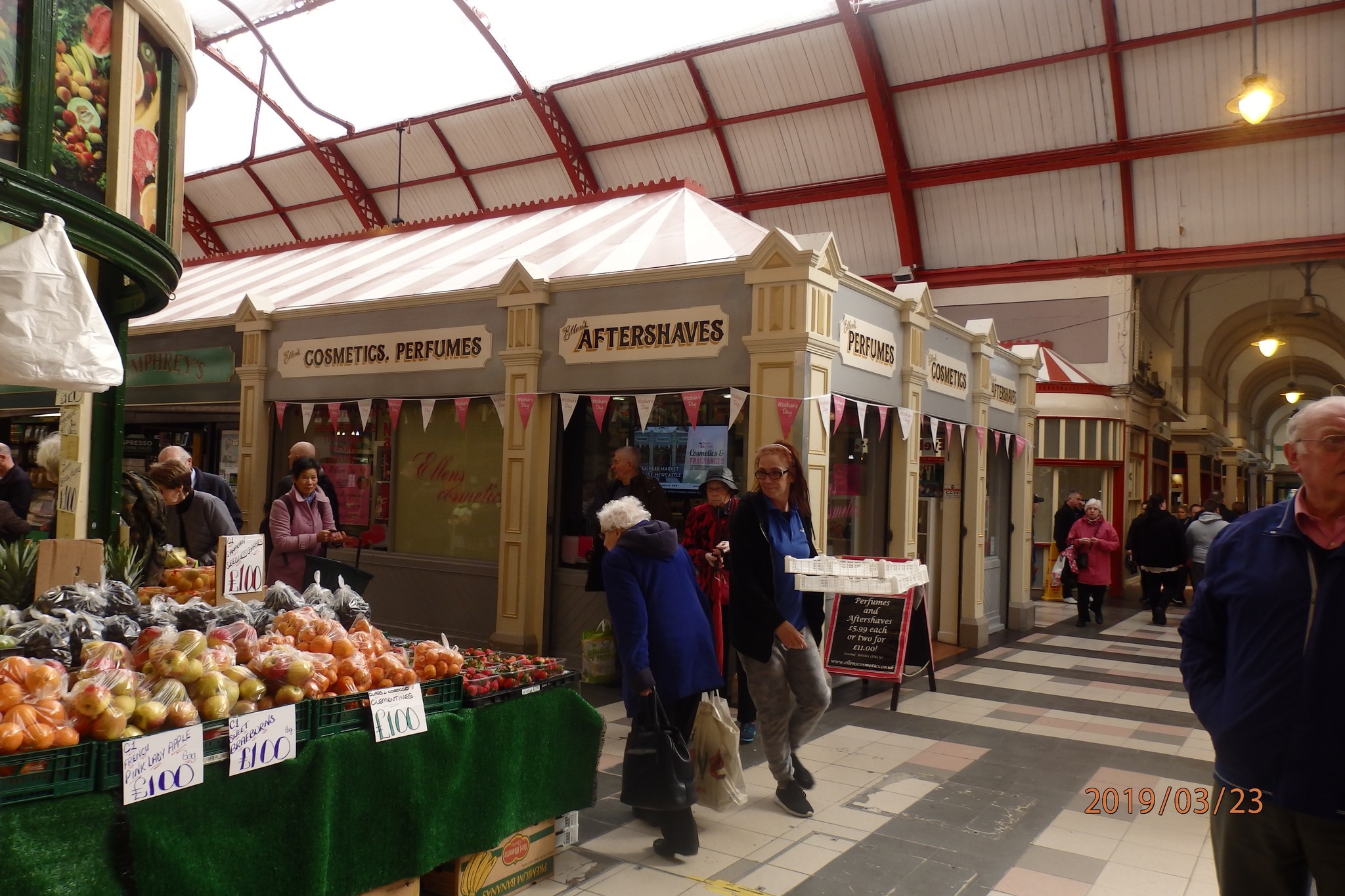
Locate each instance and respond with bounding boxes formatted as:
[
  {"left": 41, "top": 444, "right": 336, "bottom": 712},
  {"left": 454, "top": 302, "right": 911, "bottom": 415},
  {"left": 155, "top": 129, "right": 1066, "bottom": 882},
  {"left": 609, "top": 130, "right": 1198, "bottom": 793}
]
[{"left": 302, "top": 530, "right": 374, "bottom": 598}]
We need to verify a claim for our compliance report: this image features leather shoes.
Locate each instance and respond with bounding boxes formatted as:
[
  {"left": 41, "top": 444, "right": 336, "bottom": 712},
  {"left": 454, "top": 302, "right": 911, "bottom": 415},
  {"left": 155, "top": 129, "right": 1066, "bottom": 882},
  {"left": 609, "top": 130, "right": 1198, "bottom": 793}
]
[
  {"left": 1095, "top": 610, "right": 1103, "bottom": 624},
  {"left": 1076, "top": 616, "right": 1085, "bottom": 627}
]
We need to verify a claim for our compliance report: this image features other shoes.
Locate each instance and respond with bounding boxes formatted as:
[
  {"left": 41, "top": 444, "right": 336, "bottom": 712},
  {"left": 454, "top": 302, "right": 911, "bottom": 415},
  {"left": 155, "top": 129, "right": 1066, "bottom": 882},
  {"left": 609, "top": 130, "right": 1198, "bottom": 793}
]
[
  {"left": 631, "top": 805, "right": 659, "bottom": 827},
  {"left": 1150, "top": 598, "right": 1165, "bottom": 625},
  {"left": 1172, "top": 597, "right": 1186, "bottom": 605},
  {"left": 652, "top": 838, "right": 698, "bottom": 856},
  {"left": 1140, "top": 598, "right": 1146, "bottom": 601}
]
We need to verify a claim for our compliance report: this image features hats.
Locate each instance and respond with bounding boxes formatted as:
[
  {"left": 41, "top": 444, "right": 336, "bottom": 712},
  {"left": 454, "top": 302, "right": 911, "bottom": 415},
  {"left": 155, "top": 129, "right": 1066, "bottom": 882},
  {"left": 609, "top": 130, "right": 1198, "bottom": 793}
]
[
  {"left": 698, "top": 466, "right": 739, "bottom": 495},
  {"left": 1033, "top": 494, "right": 1044, "bottom": 503}
]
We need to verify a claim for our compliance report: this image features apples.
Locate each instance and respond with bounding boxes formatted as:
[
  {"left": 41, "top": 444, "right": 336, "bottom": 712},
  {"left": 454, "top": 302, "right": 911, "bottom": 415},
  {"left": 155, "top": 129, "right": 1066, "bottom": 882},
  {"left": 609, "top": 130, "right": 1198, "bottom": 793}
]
[{"left": 45, "top": 622, "right": 315, "bottom": 741}]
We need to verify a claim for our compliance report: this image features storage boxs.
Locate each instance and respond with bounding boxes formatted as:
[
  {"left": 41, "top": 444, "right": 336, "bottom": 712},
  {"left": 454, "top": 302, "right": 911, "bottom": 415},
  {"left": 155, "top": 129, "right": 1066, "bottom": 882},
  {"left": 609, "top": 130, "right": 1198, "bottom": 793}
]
[
  {"left": 785, "top": 555, "right": 929, "bottom": 594},
  {"left": 0, "top": 668, "right": 584, "bottom": 806},
  {"left": 357, "top": 810, "right": 579, "bottom": 896}
]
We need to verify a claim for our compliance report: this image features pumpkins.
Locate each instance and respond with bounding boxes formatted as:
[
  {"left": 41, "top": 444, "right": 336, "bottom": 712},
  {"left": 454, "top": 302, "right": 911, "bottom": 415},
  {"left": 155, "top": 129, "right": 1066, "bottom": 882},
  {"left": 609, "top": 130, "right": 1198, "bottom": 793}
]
[{"left": 0, "top": 85, "right": 22, "bottom": 105}]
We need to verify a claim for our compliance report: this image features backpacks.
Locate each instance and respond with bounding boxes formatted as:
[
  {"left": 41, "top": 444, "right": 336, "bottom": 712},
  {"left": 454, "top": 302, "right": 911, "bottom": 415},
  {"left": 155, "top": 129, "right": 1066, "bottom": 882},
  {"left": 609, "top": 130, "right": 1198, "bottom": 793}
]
[{"left": 260, "top": 495, "right": 323, "bottom": 562}]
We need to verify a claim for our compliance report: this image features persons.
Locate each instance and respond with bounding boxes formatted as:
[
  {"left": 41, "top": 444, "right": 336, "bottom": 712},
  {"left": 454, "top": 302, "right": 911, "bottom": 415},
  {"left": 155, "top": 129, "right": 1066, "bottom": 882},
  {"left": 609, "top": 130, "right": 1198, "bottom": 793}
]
[
  {"left": 1124, "top": 500, "right": 1151, "bottom": 611},
  {"left": 1127, "top": 493, "right": 1189, "bottom": 626},
  {"left": 0, "top": 497, "right": 32, "bottom": 551},
  {"left": 1066, "top": 498, "right": 1120, "bottom": 628},
  {"left": 585, "top": 445, "right": 674, "bottom": 683},
  {"left": 1173, "top": 504, "right": 1191, "bottom": 607},
  {"left": 34, "top": 430, "right": 61, "bottom": 538},
  {"left": 1030, "top": 493, "right": 1044, "bottom": 600},
  {"left": 148, "top": 458, "right": 240, "bottom": 588},
  {"left": 259, "top": 442, "right": 343, "bottom": 581},
  {"left": 1176, "top": 395, "right": 1345, "bottom": 896},
  {"left": 728, "top": 440, "right": 831, "bottom": 818},
  {"left": 158, "top": 445, "right": 244, "bottom": 534},
  {"left": 0, "top": 442, "right": 34, "bottom": 521},
  {"left": 1053, "top": 489, "right": 1093, "bottom": 603},
  {"left": 681, "top": 465, "right": 756, "bottom": 741},
  {"left": 265, "top": 456, "right": 346, "bottom": 596},
  {"left": 1185, "top": 499, "right": 1232, "bottom": 601},
  {"left": 595, "top": 495, "right": 723, "bottom": 859},
  {"left": 1185, "top": 492, "right": 1252, "bottom": 575}
]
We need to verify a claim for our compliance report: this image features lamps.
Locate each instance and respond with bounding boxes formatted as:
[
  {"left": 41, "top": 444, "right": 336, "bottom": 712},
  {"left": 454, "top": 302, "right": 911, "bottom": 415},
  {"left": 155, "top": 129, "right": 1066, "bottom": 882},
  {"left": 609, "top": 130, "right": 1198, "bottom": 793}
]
[
  {"left": 1251, "top": 271, "right": 1286, "bottom": 358},
  {"left": 1279, "top": 335, "right": 1305, "bottom": 404},
  {"left": 1224, "top": 0, "right": 1286, "bottom": 127}
]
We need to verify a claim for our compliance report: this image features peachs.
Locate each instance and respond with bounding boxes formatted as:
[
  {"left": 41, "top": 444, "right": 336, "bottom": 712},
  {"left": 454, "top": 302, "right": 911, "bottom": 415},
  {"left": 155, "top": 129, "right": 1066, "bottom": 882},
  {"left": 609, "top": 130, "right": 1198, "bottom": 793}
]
[
  {"left": 61, "top": 109, "right": 104, "bottom": 169},
  {"left": 56, "top": 38, "right": 93, "bottom": 104}
]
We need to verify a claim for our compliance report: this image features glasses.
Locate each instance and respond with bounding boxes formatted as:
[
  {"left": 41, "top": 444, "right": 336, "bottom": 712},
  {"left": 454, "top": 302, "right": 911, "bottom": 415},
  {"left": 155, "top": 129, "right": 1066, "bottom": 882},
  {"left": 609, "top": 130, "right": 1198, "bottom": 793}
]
[
  {"left": 755, "top": 469, "right": 789, "bottom": 479},
  {"left": 599, "top": 526, "right": 616, "bottom": 540},
  {"left": 1295, "top": 435, "right": 1345, "bottom": 450},
  {"left": 704, "top": 484, "right": 727, "bottom": 492}
]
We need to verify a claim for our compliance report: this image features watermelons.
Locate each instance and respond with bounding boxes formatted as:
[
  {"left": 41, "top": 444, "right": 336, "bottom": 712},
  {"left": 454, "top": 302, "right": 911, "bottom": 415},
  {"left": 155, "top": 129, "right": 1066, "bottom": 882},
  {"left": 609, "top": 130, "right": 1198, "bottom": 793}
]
[{"left": 79, "top": 3, "right": 112, "bottom": 60}]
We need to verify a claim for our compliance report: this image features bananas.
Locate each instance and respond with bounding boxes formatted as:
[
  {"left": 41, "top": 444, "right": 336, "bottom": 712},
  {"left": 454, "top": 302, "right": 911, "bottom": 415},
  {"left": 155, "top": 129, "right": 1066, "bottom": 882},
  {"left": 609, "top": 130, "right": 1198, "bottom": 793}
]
[
  {"left": 62, "top": 41, "right": 98, "bottom": 85},
  {"left": 134, "top": 70, "right": 160, "bottom": 134},
  {"left": 156, "top": 546, "right": 186, "bottom": 568}
]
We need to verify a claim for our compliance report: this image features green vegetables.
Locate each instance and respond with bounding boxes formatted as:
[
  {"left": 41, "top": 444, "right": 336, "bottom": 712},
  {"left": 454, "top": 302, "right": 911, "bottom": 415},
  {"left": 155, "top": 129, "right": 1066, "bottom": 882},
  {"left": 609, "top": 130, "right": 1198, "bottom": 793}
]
[
  {"left": 0, "top": 32, "right": 24, "bottom": 91},
  {"left": 50, "top": 142, "right": 84, "bottom": 181},
  {"left": 55, "top": 0, "right": 100, "bottom": 45}
]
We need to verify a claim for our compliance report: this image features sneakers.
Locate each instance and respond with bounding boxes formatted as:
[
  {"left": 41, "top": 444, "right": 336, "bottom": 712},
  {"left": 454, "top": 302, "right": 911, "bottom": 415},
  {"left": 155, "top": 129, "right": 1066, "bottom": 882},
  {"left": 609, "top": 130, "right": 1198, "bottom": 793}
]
[
  {"left": 739, "top": 721, "right": 756, "bottom": 742},
  {"left": 789, "top": 753, "right": 814, "bottom": 790},
  {"left": 1064, "top": 597, "right": 1077, "bottom": 604},
  {"left": 774, "top": 779, "right": 814, "bottom": 816}
]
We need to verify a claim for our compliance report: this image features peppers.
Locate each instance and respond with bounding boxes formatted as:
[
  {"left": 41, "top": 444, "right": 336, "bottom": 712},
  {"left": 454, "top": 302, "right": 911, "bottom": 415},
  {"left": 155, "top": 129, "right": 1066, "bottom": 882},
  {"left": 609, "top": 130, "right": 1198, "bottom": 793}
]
[
  {"left": 0, "top": 0, "right": 18, "bottom": 41},
  {"left": 0, "top": 102, "right": 22, "bottom": 124}
]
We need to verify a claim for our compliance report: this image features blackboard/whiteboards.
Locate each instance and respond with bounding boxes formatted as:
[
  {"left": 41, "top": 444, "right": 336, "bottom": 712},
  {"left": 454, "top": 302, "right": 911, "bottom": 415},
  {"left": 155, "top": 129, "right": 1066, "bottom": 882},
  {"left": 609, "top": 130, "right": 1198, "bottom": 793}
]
[{"left": 826, "top": 554, "right": 936, "bottom": 682}]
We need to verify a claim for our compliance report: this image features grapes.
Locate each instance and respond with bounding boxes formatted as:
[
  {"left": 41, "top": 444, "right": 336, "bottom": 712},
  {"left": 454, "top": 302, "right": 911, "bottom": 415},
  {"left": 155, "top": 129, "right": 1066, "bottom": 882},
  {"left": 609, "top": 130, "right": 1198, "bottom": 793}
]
[
  {"left": 7, "top": 581, "right": 371, "bottom": 670},
  {"left": 80, "top": 133, "right": 106, "bottom": 194}
]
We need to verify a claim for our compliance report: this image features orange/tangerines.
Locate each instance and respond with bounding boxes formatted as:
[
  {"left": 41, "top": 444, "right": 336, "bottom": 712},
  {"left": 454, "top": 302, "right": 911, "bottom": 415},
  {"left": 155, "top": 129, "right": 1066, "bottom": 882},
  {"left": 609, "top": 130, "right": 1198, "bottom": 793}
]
[
  {"left": 134, "top": 58, "right": 156, "bottom": 233},
  {"left": 0, "top": 654, "right": 80, "bottom": 777},
  {"left": 138, "top": 566, "right": 217, "bottom": 604},
  {"left": 412, "top": 641, "right": 463, "bottom": 695},
  {"left": 257, "top": 607, "right": 418, "bottom": 710}
]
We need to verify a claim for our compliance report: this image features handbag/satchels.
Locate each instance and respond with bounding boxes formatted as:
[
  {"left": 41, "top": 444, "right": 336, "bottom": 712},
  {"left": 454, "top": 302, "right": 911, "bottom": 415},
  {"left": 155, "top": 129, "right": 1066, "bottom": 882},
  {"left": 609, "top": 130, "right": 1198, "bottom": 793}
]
[
  {"left": 1075, "top": 553, "right": 1087, "bottom": 567},
  {"left": 620, "top": 685, "right": 697, "bottom": 811},
  {"left": 582, "top": 619, "right": 620, "bottom": 684},
  {"left": 687, "top": 689, "right": 748, "bottom": 813},
  {"left": 1124, "top": 554, "right": 1137, "bottom": 574},
  {"left": 1051, "top": 556, "right": 1066, "bottom": 586}
]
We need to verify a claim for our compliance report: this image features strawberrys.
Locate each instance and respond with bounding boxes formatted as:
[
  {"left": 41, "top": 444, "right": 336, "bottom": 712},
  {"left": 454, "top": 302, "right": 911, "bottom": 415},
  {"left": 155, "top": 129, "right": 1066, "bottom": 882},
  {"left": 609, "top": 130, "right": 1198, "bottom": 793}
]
[{"left": 458, "top": 648, "right": 564, "bottom": 696}]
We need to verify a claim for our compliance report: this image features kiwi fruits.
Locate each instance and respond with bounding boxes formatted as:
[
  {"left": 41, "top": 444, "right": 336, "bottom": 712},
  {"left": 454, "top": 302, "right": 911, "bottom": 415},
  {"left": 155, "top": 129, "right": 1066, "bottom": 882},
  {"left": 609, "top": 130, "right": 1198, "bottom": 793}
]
[{"left": 139, "top": 41, "right": 156, "bottom": 69}]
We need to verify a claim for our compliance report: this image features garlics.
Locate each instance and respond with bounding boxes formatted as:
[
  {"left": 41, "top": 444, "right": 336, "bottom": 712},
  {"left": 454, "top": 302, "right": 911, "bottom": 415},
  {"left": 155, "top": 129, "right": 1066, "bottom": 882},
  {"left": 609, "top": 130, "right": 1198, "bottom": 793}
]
[{"left": 0, "top": 120, "right": 20, "bottom": 135}]
